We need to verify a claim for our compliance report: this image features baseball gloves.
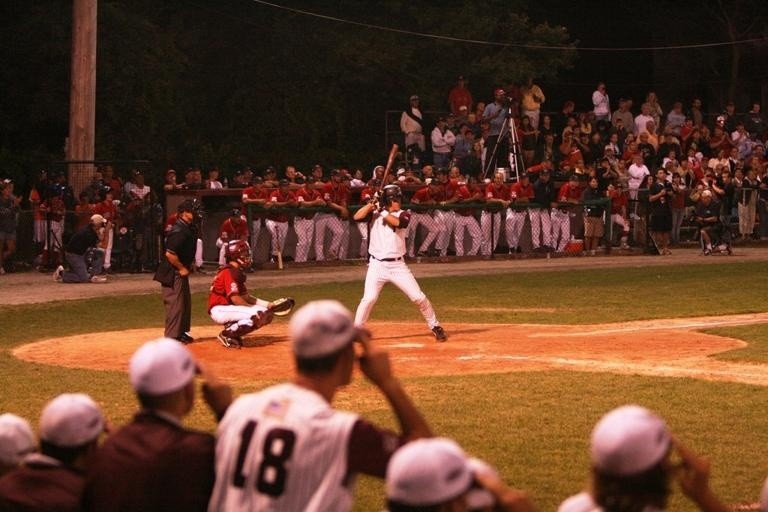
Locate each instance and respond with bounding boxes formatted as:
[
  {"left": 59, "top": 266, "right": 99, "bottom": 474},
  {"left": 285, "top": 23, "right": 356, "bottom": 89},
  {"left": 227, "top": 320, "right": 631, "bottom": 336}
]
[{"left": 273, "top": 298, "right": 295, "bottom": 315}]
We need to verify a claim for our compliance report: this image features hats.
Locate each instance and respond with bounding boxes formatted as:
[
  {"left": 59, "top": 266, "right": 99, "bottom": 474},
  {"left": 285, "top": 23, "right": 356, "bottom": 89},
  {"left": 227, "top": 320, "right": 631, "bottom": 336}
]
[
  {"left": 231, "top": 208, "right": 242, "bottom": 216},
  {"left": 569, "top": 175, "right": 580, "bottom": 182},
  {"left": 538, "top": 169, "right": 550, "bottom": 175},
  {"left": 410, "top": 95, "right": 419, "bottom": 101},
  {"left": 233, "top": 165, "right": 353, "bottom": 186},
  {"left": 89, "top": 213, "right": 108, "bottom": 226},
  {"left": 166, "top": 168, "right": 177, "bottom": 175},
  {"left": 0, "top": 183, "right": 7, "bottom": 191},
  {"left": 615, "top": 184, "right": 624, "bottom": 190},
  {"left": 208, "top": 166, "right": 218, "bottom": 172},
  {"left": 184, "top": 167, "right": 194, "bottom": 174},
  {"left": 717, "top": 116, "right": 724, "bottom": 121},
  {"left": 127, "top": 335, "right": 207, "bottom": 398},
  {"left": 436, "top": 106, "right": 468, "bottom": 122},
  {"left": 286, "top": 294, "right": 372, "bottom": 363},
  {"left": 4, "top": 179, "right": 16, "bottom": 184},
  {"left": 0, "top": 410, "right": 35, "bottom": 463},
  {"left": 588, "top": 400, "right": 680, "bottom": 479},
  {"left": 35, "top": 389, "right": 110, "bottom": 451},
  {"left": 379, "top": 432, "right": 502, "bottom": 506},
  {"left": 495, "top": 89, "right": 506, "bottom": 96},
  {"left": 135, "top": 168, "right": 144, "bottom": 175},
  {"left": 520, "top": 172, "right": 529, "bottom": 178},
  {"left": 600, "top": 157, "right": 609, "bottom": 162},
  {"left": 458, "top": 76, "right": 465, "bottom": 80}
]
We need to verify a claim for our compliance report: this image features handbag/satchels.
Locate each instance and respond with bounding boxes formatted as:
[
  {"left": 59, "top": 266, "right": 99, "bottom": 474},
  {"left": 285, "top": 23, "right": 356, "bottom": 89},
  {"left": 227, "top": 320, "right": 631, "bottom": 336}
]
[{"left": 152, "top": 261, "right": 176, "bottom": 288}]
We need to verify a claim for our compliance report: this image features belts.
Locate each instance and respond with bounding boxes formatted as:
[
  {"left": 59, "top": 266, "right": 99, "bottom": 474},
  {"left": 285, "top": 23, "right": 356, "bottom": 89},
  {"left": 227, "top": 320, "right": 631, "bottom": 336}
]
[{"left": 369, "top": 252, "right": 405, "bottom": 263}]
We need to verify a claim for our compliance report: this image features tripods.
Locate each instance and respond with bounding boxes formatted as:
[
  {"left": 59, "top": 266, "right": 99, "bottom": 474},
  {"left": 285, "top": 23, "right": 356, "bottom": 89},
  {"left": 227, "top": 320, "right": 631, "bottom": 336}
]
[{"left": 482, "top": 117, "right": 527, "bottom": 183}]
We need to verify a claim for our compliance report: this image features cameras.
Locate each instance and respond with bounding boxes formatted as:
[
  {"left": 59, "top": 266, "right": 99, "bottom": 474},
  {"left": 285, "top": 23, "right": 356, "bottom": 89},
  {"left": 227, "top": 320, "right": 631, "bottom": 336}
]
[{"left": 502, "top": 94, "right": 514, "bottom": 104}]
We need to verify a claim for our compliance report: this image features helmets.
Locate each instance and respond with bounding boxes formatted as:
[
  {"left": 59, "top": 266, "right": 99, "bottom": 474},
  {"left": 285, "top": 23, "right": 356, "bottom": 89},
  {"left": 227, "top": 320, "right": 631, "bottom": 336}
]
[
  {"left": 177, "top": 201, "right": 194, "bottom": 213},
  {"left": 49, "top": 183, "right": 65, "bottom": 196},
  {"left": 99, "top": 186, "right": 111, "bottom": 197},
  {"left": 224, "top": 238, "right": 252, "bottom": 258},
  {"left": 381, "top": 184, "right": 403, "bottom": 199},
  {"left": 435, "top": 167, "right": 449, "bottom": 173},
  {"left": 702, "top": 190, "right": 712, "bottom": 197},
  {"left": 39, "top": 168, "right": 48, "bottom": 176}
]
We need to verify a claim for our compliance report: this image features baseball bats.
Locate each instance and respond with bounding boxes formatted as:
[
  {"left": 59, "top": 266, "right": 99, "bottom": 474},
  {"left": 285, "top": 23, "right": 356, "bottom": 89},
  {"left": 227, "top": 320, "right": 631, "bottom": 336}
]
[{"left": 372, "top": 143, "right": 398, "bottom": 205}]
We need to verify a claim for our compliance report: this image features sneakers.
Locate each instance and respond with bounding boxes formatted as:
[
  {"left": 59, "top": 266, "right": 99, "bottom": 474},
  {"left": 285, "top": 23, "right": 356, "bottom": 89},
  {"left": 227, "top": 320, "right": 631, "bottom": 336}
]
[
  {"left": 89, "top": 275, "right": 107, "bottom": 284},
  {"left": 431, "top": 325, "right": 449, "bottom": 343},
  {"left": 217, "top": 321, "right": 244, "bottom": 350},
  {"left": 169, "top": 330, "right": 195, "bottom": 346},
  {"left": 53, "top": 265, "right": 64, "bottom": 282}
]
